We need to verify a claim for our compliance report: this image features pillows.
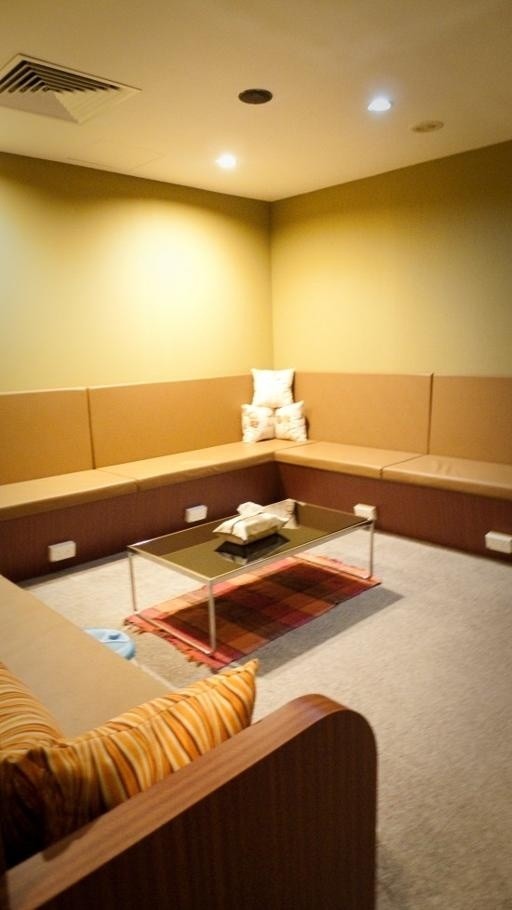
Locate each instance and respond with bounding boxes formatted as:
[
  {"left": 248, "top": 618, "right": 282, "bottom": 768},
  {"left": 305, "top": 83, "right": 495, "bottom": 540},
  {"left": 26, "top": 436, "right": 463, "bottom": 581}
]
[
  {"left": 250, "top": 370, "right": 296, "bottom": 405},
  {"left": 2, "top": 647, "right": 261, "bottom": 849},
  {"left": 1, "top": 665, "right": 60, "bottom": 867},
  {"left": 270, "top": 402, "right": 310, "bottom": 445},
  {"left": 239, "top": 403, "right": 273, "bottom": 445}
]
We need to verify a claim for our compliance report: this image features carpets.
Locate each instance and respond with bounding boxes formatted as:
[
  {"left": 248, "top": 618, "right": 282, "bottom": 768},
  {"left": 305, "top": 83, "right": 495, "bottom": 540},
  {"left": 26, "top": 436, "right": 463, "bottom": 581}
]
[{"left": 121, "top": 554, "right": 378, "bottom": 672}]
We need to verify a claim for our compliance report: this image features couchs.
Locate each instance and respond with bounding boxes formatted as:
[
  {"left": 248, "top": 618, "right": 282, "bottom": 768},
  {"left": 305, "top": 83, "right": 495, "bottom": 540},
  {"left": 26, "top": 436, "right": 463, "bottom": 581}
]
[
  {"left": 1, "top": 565, "right": 376, "bottom": 907},
  {"left": 3, "top": 370, "right": 512, "bottom": 577}
]
